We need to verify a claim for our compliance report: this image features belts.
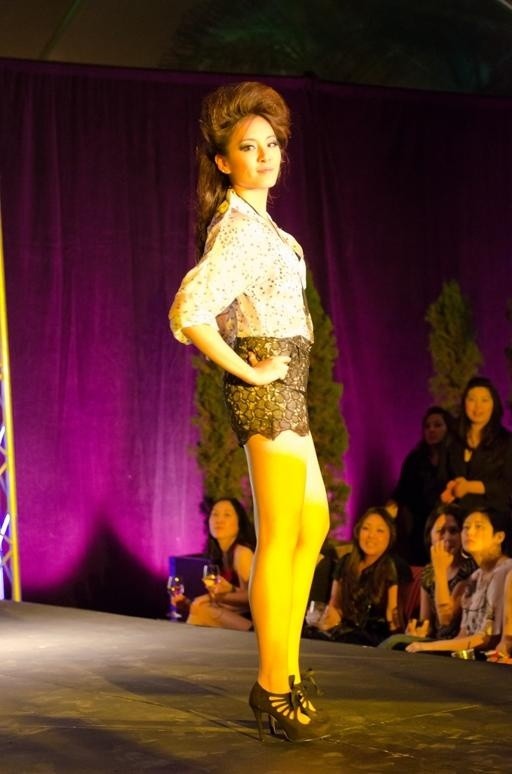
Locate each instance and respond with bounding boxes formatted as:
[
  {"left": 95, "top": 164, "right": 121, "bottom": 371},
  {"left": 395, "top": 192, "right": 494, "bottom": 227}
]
[
  {"left": 203, "top": 565, "right": 220, "bottom": 610},
  {"left": 306, "top": 601, "right": 327, "bottom": 633},
  {"left": 165, "top": 577, "right": 185, "bottom": 617}
]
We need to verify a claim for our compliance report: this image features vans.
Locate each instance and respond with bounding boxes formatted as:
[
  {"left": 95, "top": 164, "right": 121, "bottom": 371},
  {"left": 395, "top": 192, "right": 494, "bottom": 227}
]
[{"left": 250, "top": 667, "right": 331, "bottom": 743}]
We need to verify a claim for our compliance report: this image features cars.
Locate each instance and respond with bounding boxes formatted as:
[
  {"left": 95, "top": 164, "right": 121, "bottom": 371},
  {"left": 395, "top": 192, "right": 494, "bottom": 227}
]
[{"left": 452, "top": 649, "right": 476, "bottom": 663}]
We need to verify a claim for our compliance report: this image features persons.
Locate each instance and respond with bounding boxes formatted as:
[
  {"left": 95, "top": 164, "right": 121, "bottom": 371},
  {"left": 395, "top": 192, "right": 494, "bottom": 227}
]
[
  {"left": 300, "top": 540, "right": 339, "bottom": 641},
  {"left": 487, "top": 569, "right": 512, "bottom": 663},
  {"left": 167, "top": 81, "right": 337, "bottom": 744},
  {"left": 171, "top": 496, "right": 256, "bottom": 630},
  {"left": 377, "top": 503, "right": 475, "bottom": 656},
  {"left": 385, "top": 405, "right": 459, "bottom": 621},
  {"left": 435, "top": 376, "right": 511, "bottom": 558},
  {"left": 405, "top": 501, "right": 510, "bottom": 660},
  {"left": 306, "top": 506, "right": 414, "bottom": 647}
]
